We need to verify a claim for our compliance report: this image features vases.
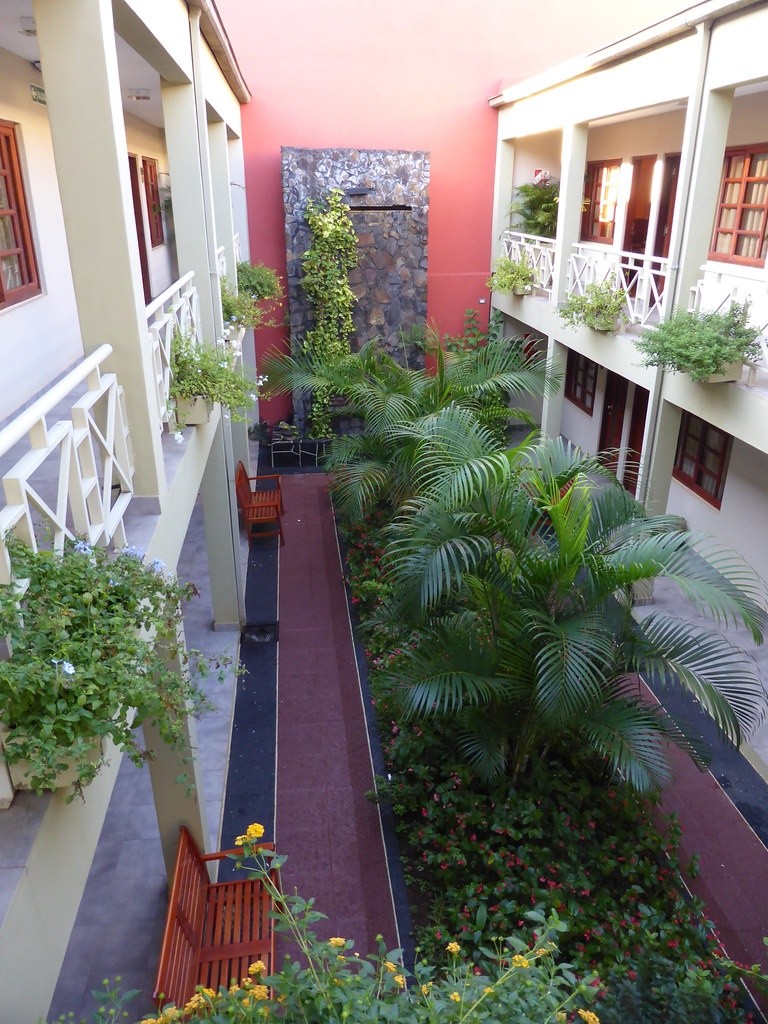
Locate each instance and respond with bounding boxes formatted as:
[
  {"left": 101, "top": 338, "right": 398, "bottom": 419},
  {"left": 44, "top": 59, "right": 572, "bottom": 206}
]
[
  {"left": 0, "top": 717, "right": 108, "bottom": 787},
  {"left": 175, "top": 394, "right": 215, "bottom": 424}
]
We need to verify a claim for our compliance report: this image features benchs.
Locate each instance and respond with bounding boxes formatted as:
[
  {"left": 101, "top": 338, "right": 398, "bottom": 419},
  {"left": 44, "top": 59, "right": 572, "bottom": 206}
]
[
  {"left": 236, "top": 462, "right": 284, "bottom": 546},
  {"left": 148, "top": 824, "right": 286, "bottom": 1024}
]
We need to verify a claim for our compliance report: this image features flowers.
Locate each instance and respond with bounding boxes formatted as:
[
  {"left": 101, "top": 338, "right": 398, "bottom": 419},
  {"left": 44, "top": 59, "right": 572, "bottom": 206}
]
[
  {"left": 0, "top": 518, "right": 252, "bottom": 800},
  {"left": 163, "top": 306, "right": 272, "bottom": 439},
  {"left": 219, "top": 272, "right": 295, "bottom": 334},
  {"left": 236, "top": 258, "right": 287, "bottom": 307}
]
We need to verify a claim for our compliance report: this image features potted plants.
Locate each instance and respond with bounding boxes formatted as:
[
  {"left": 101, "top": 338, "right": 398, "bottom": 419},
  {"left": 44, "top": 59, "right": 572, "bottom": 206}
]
[
  {"left": 485, "top": 250, "right": 541, "bottom": 295},
  {"left": 556, "top": 271, "right": 631, "bottom": 332},
  {"left": 631, "top": 293, "right": 768, "bottom": 384}
]
